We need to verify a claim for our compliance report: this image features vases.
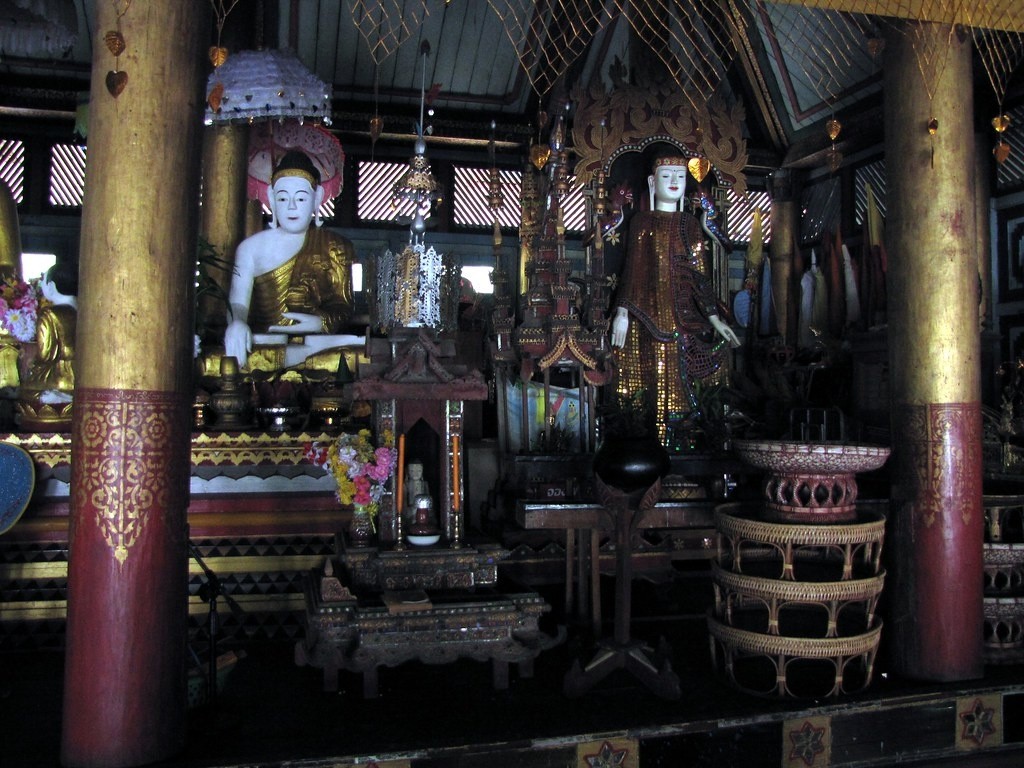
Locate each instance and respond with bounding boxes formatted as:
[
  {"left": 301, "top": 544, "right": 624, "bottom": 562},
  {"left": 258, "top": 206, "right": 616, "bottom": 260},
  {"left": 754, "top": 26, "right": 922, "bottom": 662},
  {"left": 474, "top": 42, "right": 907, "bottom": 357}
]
[{"left": 346, "top": 502, "right": 375, "bottom": 549}]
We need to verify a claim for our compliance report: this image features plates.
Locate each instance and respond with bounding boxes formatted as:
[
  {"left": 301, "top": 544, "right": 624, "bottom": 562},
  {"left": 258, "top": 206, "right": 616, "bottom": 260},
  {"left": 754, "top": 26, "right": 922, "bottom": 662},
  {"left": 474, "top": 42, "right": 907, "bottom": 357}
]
[{"left": 0, "top": 440, "right": 36, "bottom": 536}]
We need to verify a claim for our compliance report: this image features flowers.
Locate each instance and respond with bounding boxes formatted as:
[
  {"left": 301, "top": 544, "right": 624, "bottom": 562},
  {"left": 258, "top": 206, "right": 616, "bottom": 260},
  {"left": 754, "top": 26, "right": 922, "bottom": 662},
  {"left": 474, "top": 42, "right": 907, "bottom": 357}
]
[
  {"left": 0, "top": 269, "right": 52, "bottom": 344},
  {"left": 303, "top": 428, "right": 397, "bottom": 514}
]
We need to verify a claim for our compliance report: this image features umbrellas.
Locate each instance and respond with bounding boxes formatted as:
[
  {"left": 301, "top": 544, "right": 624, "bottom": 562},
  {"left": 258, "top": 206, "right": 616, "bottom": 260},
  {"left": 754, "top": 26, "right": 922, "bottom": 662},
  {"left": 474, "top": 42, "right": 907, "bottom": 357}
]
[
  {"left": 205, "top": 48, "right": 333, "bottom": 171},
  {"left": 245, "top": 118, "right": 344, "bottom": 213}
]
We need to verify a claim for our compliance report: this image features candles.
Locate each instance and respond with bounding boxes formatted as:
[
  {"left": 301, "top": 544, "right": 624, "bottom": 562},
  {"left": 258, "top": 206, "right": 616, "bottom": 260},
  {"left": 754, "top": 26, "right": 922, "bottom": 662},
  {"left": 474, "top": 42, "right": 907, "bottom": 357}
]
[
  {"left": 396, "top": 434, "right": 404, "bottom": 514},
  {"left": 453, "top": 435, "right": 459, "bottom": 510}
]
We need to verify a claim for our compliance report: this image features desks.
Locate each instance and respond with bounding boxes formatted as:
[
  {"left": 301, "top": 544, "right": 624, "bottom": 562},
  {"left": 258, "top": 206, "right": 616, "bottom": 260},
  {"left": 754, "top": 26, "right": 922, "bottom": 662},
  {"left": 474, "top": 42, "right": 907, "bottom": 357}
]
[{"left": 513, "top": 496, "right": 726, "bottom": 636}]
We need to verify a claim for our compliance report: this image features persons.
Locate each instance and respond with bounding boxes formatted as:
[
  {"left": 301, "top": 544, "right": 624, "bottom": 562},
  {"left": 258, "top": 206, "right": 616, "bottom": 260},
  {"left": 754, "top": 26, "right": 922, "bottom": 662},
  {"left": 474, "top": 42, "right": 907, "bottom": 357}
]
[
  {"left": 606, "top": 151, "right": 741, "bottom": 449},
  {"left": 402, "top": 457, "right": 437, "bottom": 525},
  {"left": 20, "top": 263, "right": 78, "bottom": 404},
  {"left": 194, "top": 150, "right": 370, "bottom": 382}
]
